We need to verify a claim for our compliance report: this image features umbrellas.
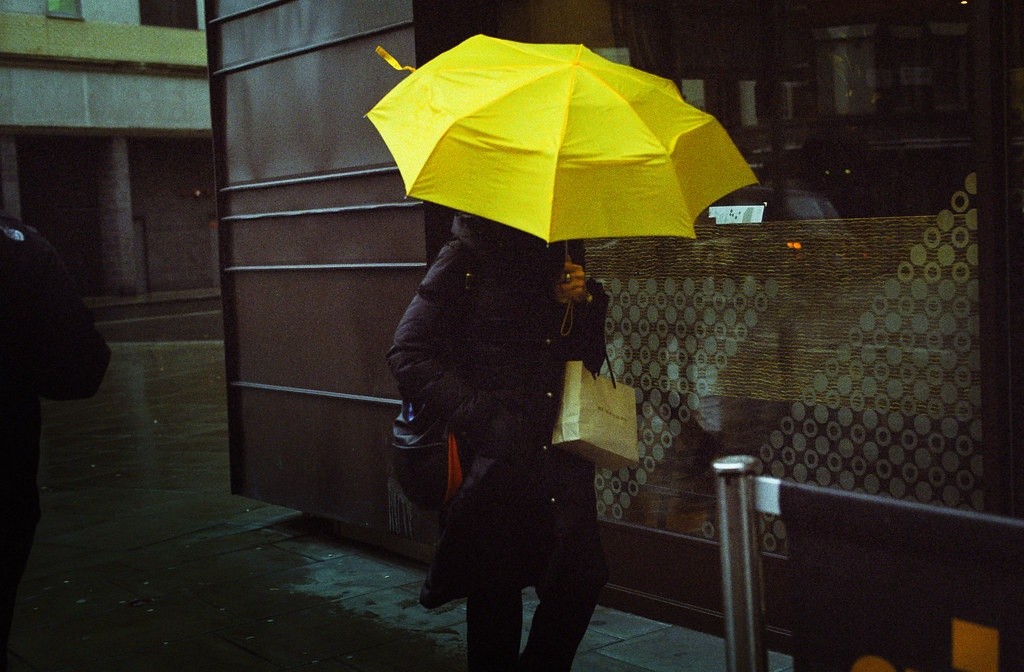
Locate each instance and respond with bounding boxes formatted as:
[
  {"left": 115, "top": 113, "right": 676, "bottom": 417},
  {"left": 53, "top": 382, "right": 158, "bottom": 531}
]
[{"left": 363, "top": 34, "right": 759, "bottom": 335}]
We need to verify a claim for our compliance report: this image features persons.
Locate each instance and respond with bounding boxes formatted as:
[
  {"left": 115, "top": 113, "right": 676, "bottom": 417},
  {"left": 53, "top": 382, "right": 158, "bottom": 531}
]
[
  {"left": 0, "top": 214, "right": 113, "bottom": 672},
  {"left": 386, "top": 211, "right": 607, "bottom": 672}
]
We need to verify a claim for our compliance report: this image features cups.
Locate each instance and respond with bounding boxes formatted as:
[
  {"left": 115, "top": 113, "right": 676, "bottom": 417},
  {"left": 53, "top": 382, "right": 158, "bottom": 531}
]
[{"left": 813, "top": 24, "right": 879, "bottom": 118}]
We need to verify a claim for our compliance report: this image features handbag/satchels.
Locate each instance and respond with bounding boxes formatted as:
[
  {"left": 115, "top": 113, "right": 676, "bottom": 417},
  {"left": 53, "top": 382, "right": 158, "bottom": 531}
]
[
  {"left": 391, "top": 399, "right": 449, "bottom": 511},
  {"left": 552, "top": 354, "right": 639, "bottom": 471}
]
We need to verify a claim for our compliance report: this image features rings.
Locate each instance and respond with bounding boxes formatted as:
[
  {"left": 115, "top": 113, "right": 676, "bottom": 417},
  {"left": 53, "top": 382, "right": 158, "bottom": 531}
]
[{"left": 565, "top": 271, "right": 571, "bottom": 283}]
